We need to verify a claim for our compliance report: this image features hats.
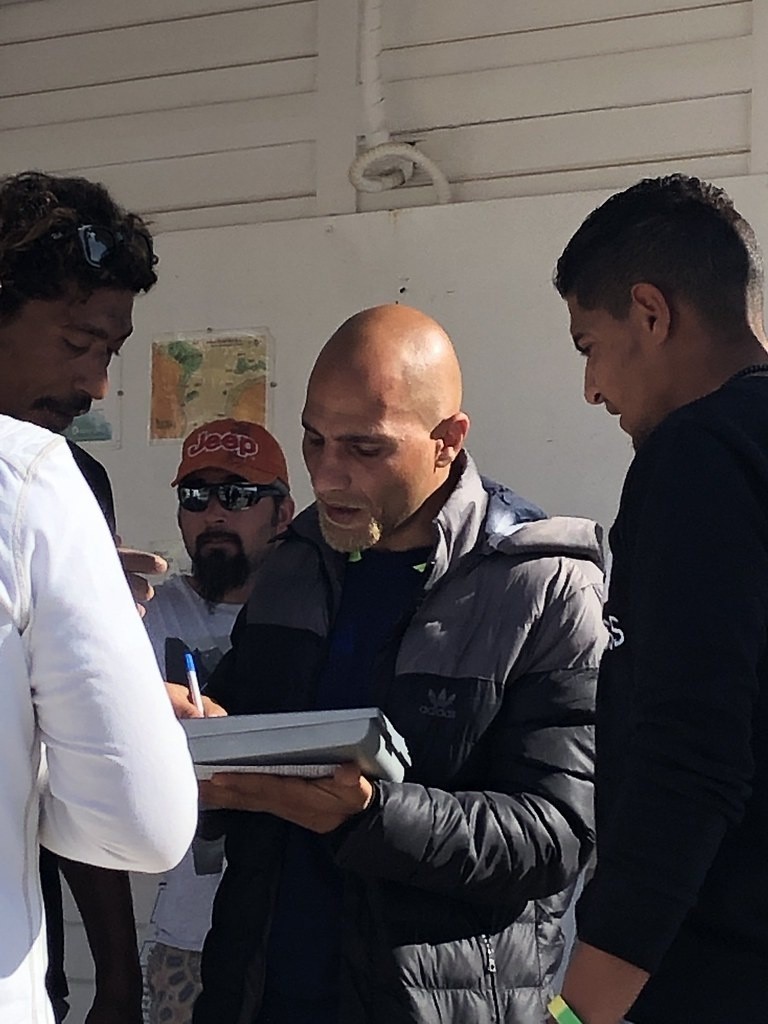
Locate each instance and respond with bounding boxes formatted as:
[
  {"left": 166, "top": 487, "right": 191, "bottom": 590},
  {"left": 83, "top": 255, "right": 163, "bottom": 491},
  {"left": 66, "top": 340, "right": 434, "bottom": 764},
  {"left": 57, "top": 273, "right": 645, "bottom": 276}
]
[{"left": 170, "top": 419, "right": 288, "bottom": 486}]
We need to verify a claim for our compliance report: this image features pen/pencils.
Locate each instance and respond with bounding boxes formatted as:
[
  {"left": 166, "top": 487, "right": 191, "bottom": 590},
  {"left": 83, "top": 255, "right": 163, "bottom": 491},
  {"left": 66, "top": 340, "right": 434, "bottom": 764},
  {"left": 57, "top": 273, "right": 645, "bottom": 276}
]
[{"left": 184, "top": 652, "right": 205, "bottom": 716}]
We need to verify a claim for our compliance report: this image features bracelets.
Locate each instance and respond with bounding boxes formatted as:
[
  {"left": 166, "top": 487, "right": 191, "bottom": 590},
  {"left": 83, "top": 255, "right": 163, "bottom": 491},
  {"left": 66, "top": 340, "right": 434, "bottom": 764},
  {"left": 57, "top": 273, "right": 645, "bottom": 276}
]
[{"left": 546, "top": 995, "right": 582, "bottom": 1024}]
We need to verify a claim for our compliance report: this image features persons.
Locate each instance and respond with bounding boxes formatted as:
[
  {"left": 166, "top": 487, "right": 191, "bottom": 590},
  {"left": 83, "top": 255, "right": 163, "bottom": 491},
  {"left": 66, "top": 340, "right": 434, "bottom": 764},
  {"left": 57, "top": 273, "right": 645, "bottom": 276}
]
[
  {"left": 142, "top": 170, "right": 767, "bottom": 1024},
  {"left": 1, "top": 163, "right": 198, "bottom": 1023}
]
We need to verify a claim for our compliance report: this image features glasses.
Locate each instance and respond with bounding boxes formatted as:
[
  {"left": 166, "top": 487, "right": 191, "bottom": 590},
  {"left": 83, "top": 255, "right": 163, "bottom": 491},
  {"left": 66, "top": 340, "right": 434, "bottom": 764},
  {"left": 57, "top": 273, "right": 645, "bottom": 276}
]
[
  {"left": 177, "top": 479, "right": 280, "bottom": 511},
  {"left": 25, "top": 223, "right": 154, "bottom": 269}
]
